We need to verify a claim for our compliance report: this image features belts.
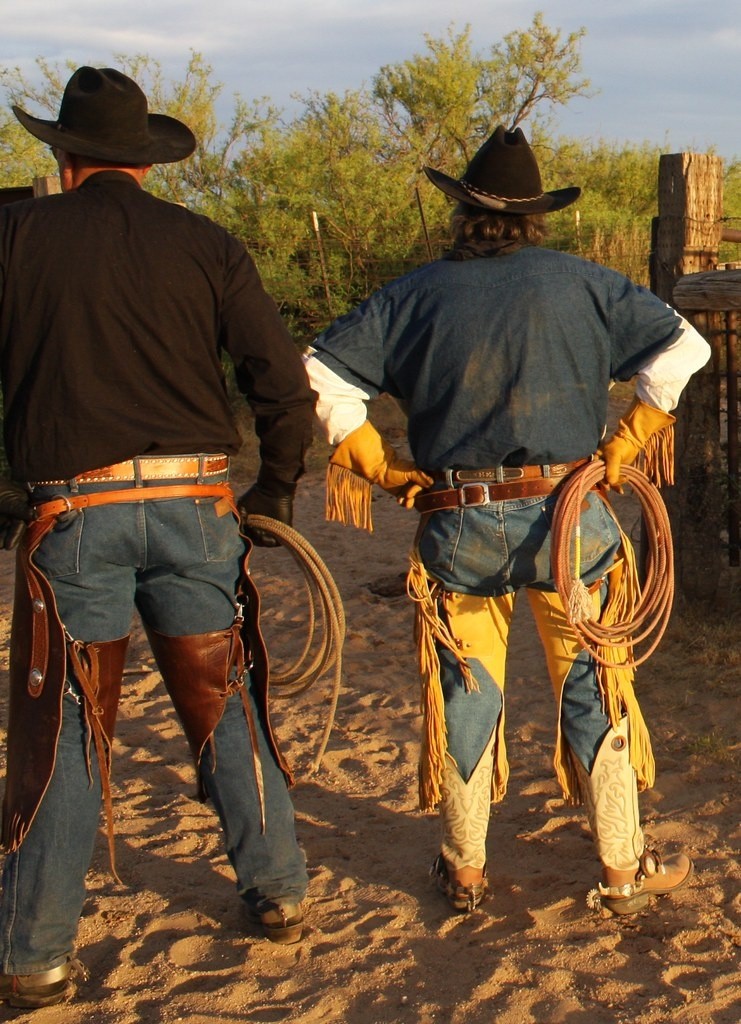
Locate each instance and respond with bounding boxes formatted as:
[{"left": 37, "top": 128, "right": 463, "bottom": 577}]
[
  {"left": 434, "top": 458, "right": 591, "bottom": 483},
  {"left": 29, "top": 483, "right": 233, "bottom": 520},
  {"left": 413, "top": 475, "right": 601, "bottom": 515},
  {"left": 30, "top": 454, "right": 227, "bottom": 486}
]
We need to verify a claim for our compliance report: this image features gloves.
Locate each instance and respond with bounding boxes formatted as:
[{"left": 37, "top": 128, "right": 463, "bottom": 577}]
[
  {"left": 0, "top": 479, "right": 37, "bottom": 551},
  {"left": 592, "top": 393, "right": 677, "bottom": 496},
  {"left": 323, "top": 419, "right": 433, "bottom": 534},
  {"left": 231, "top": 482, "right": 295, "bottom": 548}
]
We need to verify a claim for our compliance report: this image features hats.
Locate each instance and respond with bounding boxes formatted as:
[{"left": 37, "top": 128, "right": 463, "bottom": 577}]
[
  {"left": 422, "top": 124, "right": 580, "bottom": 214},
  {"left": 11, "top": 66, "right": 195, "bottom": 164}
]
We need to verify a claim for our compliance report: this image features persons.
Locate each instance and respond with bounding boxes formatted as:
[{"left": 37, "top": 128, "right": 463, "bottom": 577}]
[
  {"left": 2, "top": 68, "right": 323, "bottom": 1009},
  {"left": 299, "top": 123, "right": 716, "bottom": 915}
]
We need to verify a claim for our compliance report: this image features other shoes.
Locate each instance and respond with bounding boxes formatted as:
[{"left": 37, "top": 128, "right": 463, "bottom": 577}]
[
  {"left": 0, "top": 956, "right": 70, "bottom": 1010},
  {"left": 428, "top": 856, "right": 485, "bottom": 911},
  {"left": 250, "top": 903, "right": 303, "bottom": 945},
  {"left": 599, "top": 854, "right": 694, "bottom": 916}
]
